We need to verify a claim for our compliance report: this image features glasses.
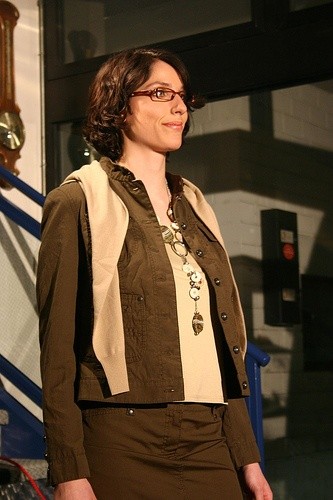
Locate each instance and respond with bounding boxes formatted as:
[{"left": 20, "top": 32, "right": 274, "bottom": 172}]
[{"left": 130, "top": 87, "right": 187, "bottom": 102}]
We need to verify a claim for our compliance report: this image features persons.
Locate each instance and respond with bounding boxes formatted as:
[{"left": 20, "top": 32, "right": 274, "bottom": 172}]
[{"left": 37, "top": 47, "right": 276, "bottom": 500}]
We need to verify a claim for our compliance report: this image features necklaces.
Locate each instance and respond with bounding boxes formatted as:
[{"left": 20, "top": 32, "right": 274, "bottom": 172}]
[{"left": 156, "top": 177, "right": 204, "bottom": 336}]
